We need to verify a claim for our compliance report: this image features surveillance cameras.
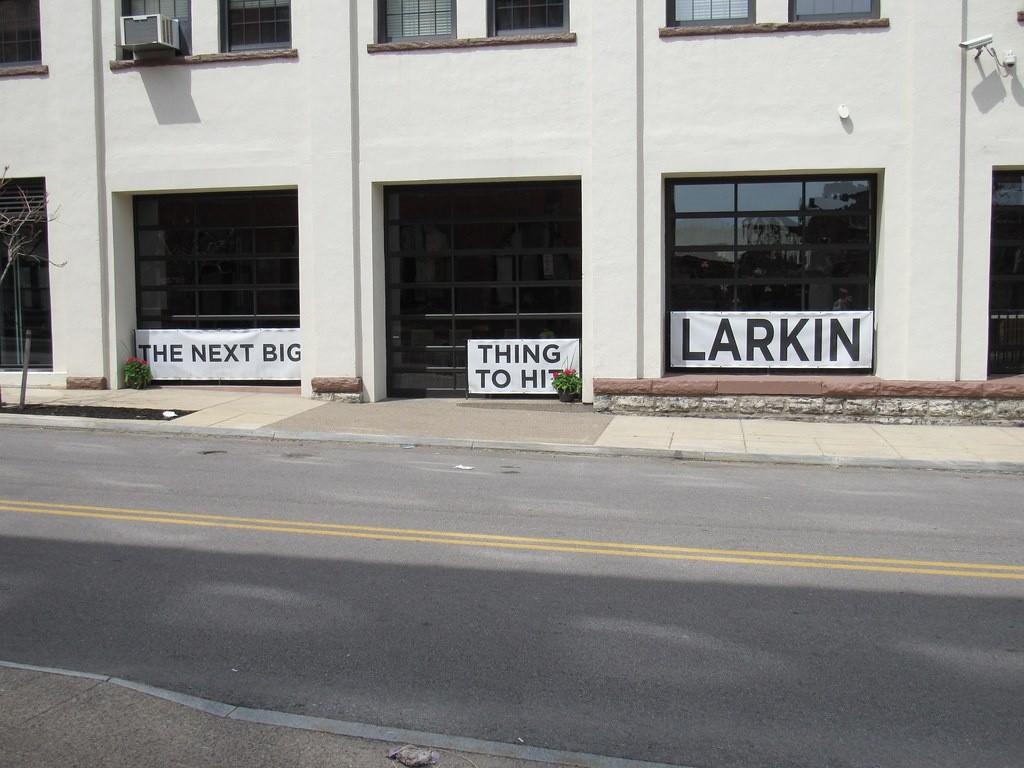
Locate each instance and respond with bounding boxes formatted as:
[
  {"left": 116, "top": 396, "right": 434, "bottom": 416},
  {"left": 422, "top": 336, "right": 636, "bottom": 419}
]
[
  {"left": 957, "top": 32, "right": 993, "bottom": 50},
  {"left": 1003, "top": 53, "right": 1016, "bottom": 69}
]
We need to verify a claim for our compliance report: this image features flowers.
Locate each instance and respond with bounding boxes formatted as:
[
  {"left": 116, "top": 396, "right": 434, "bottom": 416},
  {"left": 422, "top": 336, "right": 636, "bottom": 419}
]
[
  {"left": 123, "top": 357, "right": 153, "bottom": 388},
  {"left": 551, "top": 368, "right": 582, "bottom": 395}
]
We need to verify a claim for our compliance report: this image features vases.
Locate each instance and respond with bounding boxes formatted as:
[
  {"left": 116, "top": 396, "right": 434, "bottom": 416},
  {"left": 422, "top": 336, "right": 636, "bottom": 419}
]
[
  {"left": 558, "top": 388, "right": 575, "bottom": 402},
  {"left": 128, "top": 377, "right": 145, "bottom": 388}
]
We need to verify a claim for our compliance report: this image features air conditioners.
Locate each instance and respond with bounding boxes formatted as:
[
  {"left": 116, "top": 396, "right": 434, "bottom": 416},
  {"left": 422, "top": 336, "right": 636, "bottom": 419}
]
[{"left": 120, "top": 13, "right": 180, "bottom": 49}]
